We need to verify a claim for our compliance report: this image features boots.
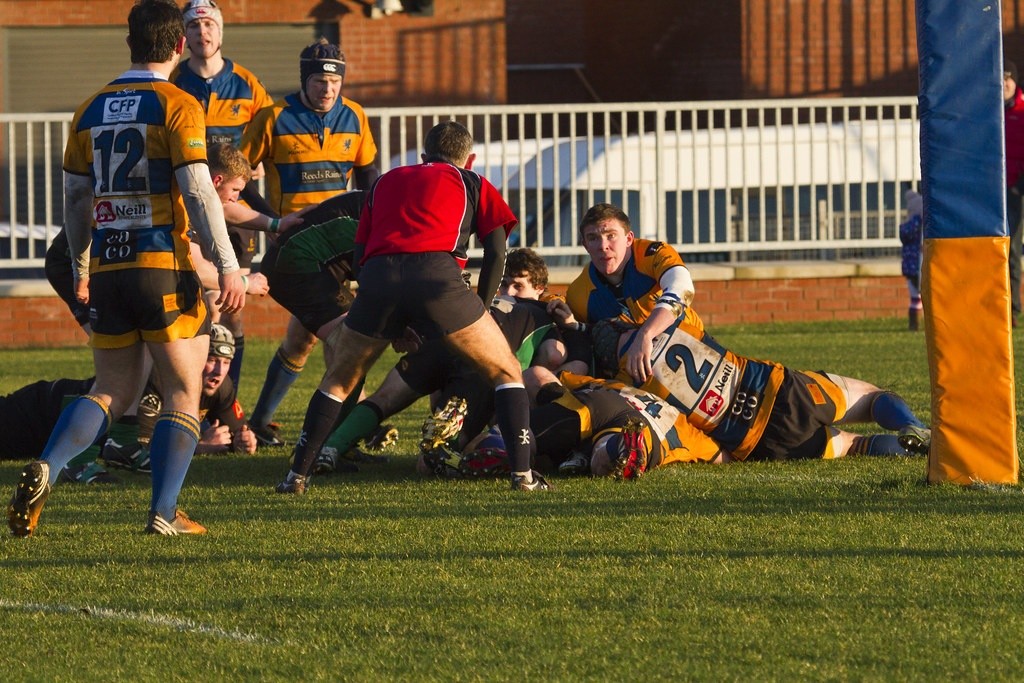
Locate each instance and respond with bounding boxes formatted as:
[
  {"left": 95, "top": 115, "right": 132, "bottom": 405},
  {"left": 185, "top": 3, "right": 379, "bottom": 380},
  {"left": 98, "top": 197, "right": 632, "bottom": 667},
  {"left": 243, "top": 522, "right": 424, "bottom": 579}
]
[{"left": 908, "top": 307, "right": 923, "bottom": 329}]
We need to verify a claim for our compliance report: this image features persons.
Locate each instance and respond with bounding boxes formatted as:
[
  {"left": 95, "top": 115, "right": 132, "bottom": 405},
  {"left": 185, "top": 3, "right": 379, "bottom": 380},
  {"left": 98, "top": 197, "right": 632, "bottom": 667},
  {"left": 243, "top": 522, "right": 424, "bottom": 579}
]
[
  {"left": 1003, "top": 59, "right": 1024, "bottom": 329},
  {"left": 263, "top": 121, "right": 552, "bottom": 494},
  {"left": 246, "top": 43, "right": 397, "bottom": 452},
  {"left": 900, "top": 189, "right": 925, "bottom": 334},
  {"left": 0, "top": 0, "right": 318, "bottom": 537},
  {"left": 312, "top": 203, "right": 933, "bottom": 481}
]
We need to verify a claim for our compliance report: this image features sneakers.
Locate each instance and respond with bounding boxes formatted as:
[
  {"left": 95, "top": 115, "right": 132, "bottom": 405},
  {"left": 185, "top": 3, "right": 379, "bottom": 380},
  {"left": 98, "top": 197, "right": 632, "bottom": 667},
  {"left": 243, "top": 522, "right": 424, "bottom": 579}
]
[
  {"left": 58, "top": 459, "right": 119, "bottom": 484},
  {"left": 897, "top": 425, "right": 932, "bottom": 453},
  {"left": 99, "top": 437, "right": 154, "bottom": 473},
  {"left": 8, "top": 461, "right": 51, "bottom": 537},
  {"left": 367, "top": 422, "right": 401, "bottom": 450},
  {"left": 314, "top": 446, "right": 336, "bottom": 474},
  {"left": 272, "top": 476, "right": 304, "bottom": 495},
  {"left": 145, "top": 508, "right": 206, "bottom": 536},
  {"left": 247, "top": 422, "right": 289, "bottom": 446},
  {"left": 462, "top": 447, "right": 509, "bottom": 477},
  {"left": 344, "top": 447, "right": 390, "bottom": 465},
  {"left": 420, "top": 437, "right": 469, "bottom": 480},
  {"left": 616, "top": 411, "right": 647, "bottom": 483},
  {"left": 556, "top": 450, "right": 589, "bottom": 477},
  {"left": 511, "top": 471, "right": 552, "bottom": 493},
  {"left": 420, "top": 395, "right": 469, "bottom": 451}
]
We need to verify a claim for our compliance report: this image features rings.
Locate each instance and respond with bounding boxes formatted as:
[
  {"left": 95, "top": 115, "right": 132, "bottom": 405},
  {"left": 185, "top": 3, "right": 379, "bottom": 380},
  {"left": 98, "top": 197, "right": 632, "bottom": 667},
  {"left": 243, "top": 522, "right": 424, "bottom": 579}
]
[{"left": 632, "top": 369, "right": 639, "bottom": 373}]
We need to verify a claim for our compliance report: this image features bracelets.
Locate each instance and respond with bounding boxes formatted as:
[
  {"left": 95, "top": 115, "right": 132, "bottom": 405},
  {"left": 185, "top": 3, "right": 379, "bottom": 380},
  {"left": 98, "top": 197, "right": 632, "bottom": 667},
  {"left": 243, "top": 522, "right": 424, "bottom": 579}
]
[
  {"left": 241, "top": 276, "right": 250, "bottom": 295},
  {"left": 577, "top": 321, "right": 583, "bottom": 332},
  {"left": 266, "top": 217, "right": 281, "bottom": 233}
]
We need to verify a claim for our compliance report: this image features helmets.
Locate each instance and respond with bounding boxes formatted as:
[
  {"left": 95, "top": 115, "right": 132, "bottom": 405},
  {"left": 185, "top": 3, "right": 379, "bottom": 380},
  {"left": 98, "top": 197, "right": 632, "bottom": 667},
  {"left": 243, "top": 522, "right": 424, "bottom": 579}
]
[{"left": 589, "top": 317, "right": 636, "bottom": 374}]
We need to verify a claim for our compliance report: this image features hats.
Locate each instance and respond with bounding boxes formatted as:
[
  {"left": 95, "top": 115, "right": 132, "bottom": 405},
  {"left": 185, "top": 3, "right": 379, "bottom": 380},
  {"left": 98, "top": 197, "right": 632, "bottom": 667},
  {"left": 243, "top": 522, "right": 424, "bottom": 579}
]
[
  {"left": 180, "top": 0, "right": 223, "bottom": 48},
  {"left": 210, "top": 321, "right": 237, "bottom": 358},
  {"left": 904, "top": 191, "right": 922, "bottom": 217},
  {"left": 300, "top": 37, "right": 345, "bottom": 93}
]
